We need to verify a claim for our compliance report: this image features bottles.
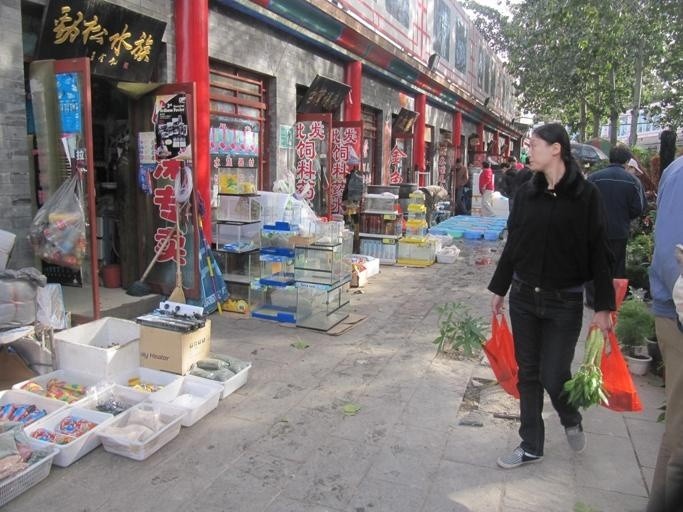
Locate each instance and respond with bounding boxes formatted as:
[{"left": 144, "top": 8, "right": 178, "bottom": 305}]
[
  {"left": 31, "top": 213, "right": 85, "bottom": 267},
  {"left": 359, "top": 214, "right": 402, "bottom": 259}
]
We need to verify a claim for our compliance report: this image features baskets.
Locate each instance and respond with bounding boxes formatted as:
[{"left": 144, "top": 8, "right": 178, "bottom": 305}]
[
  {"left": 0, "top": 437, "right": 59, "bottom": 507},
  {"left": 97, "top": 401, "right": 189, "bottom": 461}
]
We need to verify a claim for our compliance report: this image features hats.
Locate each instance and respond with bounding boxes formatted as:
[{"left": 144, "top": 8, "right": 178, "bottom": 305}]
[{"left": 628, "top": 158, "right": 644, "bottom": 175}]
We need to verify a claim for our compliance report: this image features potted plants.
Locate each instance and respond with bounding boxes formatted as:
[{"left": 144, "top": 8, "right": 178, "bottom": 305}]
[{"left": 616, "top": 263, "right": 661, "bottom": 377}]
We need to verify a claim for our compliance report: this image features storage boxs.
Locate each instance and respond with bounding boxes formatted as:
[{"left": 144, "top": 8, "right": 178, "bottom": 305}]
[
  {"left": 1, "top": 316, "right": 251, "bottom": 468},
  {"left": 359, "top": 192, "right": 460, "bottom": 268},
  {"left": 211, "top": 166, "right": 379, "bottom": 331},
  {"left": 470, "top": 172, "right": 511, "bottom": 218}
]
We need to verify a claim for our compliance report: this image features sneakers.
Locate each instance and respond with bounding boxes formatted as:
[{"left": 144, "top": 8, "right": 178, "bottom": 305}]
[
  {"left": 497, "top": 446, "right": 544, "bottom": 469},
  {"left": 564, "top": 423, "right": 586, "bottom": 453}
]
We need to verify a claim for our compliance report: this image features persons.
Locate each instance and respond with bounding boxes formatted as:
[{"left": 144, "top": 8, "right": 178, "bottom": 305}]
[
  {"left": 419, "top": 185, "right": 448, "bottom": 228},
  {"left": 584, "top": 142, "right": 648, "bottom": 310},
  {"left": 625, "top": 130, "right": 677, "bottom": 240},
  {"left": 449, "top": 156, "right": 535, "bottom": 215},
  {"left": 649, "top": 155, "right": 683, "bottom": 510},
  {"left": 488, "top": 124, "right": 617, "bottom": 469}
]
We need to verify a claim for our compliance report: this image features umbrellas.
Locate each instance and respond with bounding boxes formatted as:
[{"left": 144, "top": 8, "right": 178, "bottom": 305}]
[{"left": 568, "top": 143, "right": 608, "bottom": 162}]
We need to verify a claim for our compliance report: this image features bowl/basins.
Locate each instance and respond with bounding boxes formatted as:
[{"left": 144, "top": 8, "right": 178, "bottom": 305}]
[{"left": 428, "top": 214, "right": 508, "bottom": 241}]
